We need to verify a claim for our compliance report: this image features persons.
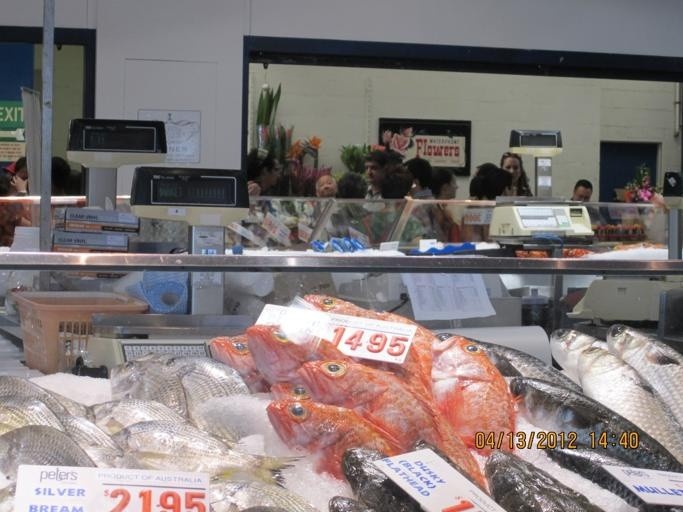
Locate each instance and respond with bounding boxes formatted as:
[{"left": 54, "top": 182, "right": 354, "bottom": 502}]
[
  {"left": 567, "top": 179, "right": 603, "bottom": 225},
  {"left": 0, "top": 154, "right": 75, "bottom": 246},
  {"left": 460, "top": 151, "right": 534, "bottom": 243},
  {"left": 244, "top": 145, "right": 460, "bottom": 247}
]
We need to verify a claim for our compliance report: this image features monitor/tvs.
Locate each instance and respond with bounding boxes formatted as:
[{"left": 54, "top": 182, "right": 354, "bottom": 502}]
[
  {"left": 307, "top": 197, "right": 335, "bottom": 249},
  {"left": 383, "top": 194, "right": 414, "bottom": 243}
]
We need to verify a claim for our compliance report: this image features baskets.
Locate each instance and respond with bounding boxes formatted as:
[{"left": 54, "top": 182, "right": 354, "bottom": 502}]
[{"left": 11, "top": 289, "right": 148, "bottom": 374}]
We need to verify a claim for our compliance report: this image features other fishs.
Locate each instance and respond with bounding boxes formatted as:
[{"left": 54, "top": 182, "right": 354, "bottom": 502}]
[{"left": 0, "top": 294, "right": 683, "bottom": 512}]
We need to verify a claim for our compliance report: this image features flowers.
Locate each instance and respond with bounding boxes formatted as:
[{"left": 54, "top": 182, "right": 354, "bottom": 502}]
[
  {"left": 257, "top": 122, "right": 387, "bottom": 181},
  {"left": 624, "top": 165, "right": 664, "bottom": 204}
]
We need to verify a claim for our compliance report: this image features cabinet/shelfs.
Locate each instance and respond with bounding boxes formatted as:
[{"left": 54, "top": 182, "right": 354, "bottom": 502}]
[{"left": 0, "top": 197, "right": 683, "bottom": 512}]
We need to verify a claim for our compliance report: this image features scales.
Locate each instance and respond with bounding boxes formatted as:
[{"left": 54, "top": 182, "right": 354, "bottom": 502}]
[
  {"left": 54, "top": 116, "right": 168, "bottom": 251},
  {"left": 488, "top": 130, "right": 594, "bottom": 243},
  {"left": 88, "top": 165, "right": 252, "bottom": 378}
]
